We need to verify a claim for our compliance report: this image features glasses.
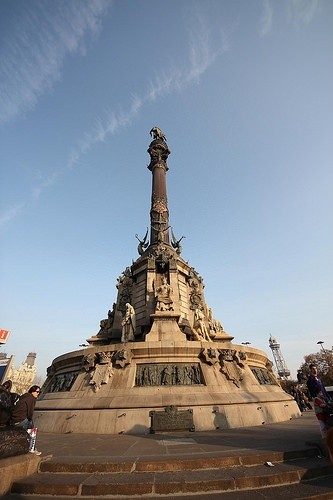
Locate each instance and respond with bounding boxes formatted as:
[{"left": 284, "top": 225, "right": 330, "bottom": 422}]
[{"left": 33, "top": 391, "right": 40, "bottom": 393}]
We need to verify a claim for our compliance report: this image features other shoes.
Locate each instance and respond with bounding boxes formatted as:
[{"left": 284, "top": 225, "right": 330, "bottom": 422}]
[{"left": 29, "top": 450, "right": 41, "bottom": 455}]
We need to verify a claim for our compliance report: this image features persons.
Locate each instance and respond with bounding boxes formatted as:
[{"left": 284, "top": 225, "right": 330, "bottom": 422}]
[
  {"left": 192, "top": 304, "right": 212, "bottom": 342},
  {"left": 9, "top": 385, "right": 41, "bottom": 455},
  {"left": 154, "top": 276, "right": 174, "bottom": 312},
  {"left": 306, "top": 364, "right": 333, "bottom": 461},
  {"left": 288, "top": 384, "right": 313, "bottom": 412},
  {"left": 0, "top": 380, "right": 20, "bottom": 426},
  {"left": 119, "top": 302, "right": 135, "bottom": 343}
]
